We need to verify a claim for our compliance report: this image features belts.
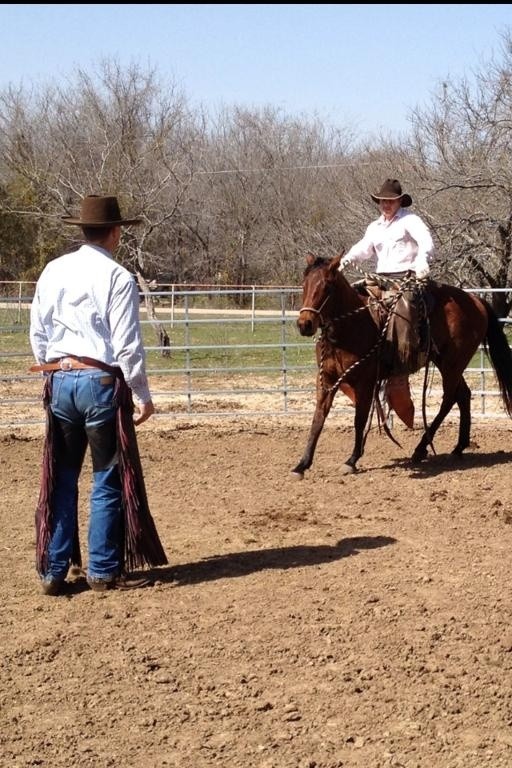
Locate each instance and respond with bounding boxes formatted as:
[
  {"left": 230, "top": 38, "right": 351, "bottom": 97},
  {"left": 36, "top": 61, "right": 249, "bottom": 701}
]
[{"left": 30, "top": 356, "right": 116, "bottom": 372}]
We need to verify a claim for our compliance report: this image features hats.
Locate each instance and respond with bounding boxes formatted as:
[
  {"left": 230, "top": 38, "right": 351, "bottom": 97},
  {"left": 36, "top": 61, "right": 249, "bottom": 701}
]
[
  {"left": 59, "top": 193, "right": 142, "bottom": 228},
  {"left": 370, "top": 177, "right": 413, "bottom": 208}
]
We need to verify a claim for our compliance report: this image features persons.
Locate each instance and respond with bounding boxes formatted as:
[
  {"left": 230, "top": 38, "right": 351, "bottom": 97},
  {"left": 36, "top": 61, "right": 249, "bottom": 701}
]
[
  {"left": 332, "top": 179, "right": 435, "bottom": 374},
  {"left": 29, "top": 195, "right": 154, "bottom": 595}
]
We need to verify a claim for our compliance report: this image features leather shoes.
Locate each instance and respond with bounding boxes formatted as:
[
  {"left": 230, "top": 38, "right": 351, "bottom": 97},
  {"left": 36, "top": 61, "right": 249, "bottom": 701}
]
[
  {"left": 40, "top": 571, "right": 62, "bottom": 595},
  {"left": 83, "top": 569, "right": 157, "bottom": 591}
]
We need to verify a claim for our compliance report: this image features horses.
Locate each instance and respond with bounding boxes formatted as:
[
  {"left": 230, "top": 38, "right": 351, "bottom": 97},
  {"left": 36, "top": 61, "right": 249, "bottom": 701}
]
[{"left": 289, "top": 247, "right": 511, "bottom": 481}]
